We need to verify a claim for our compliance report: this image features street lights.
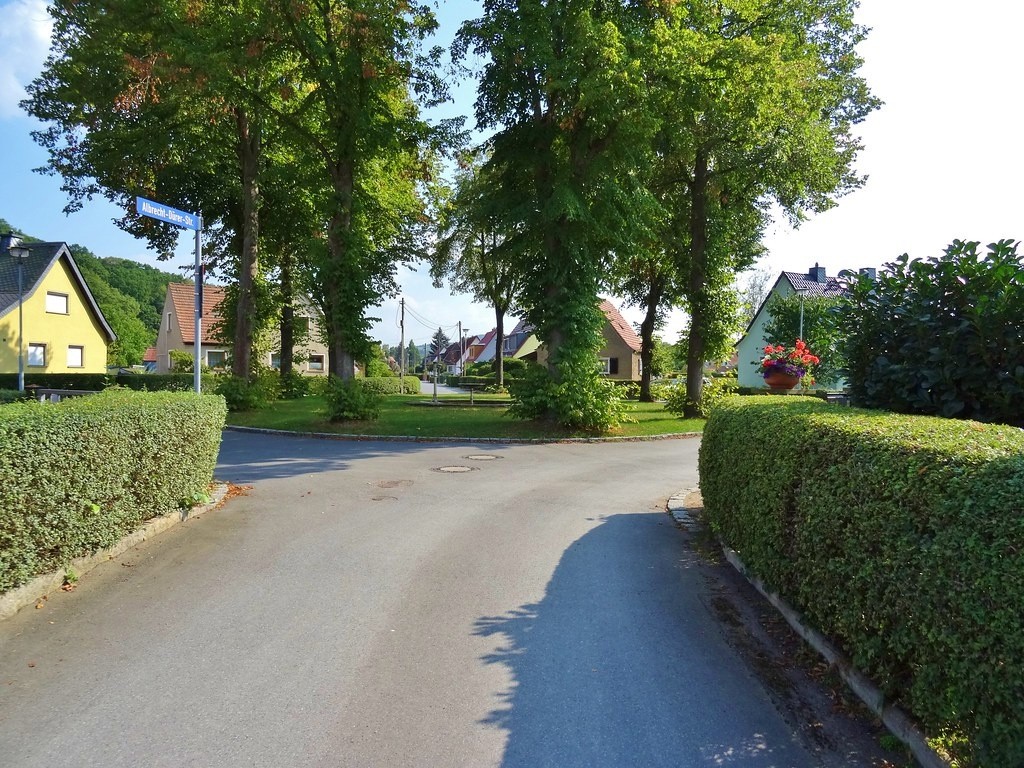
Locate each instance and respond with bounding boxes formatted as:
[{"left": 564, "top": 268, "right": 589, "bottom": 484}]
[
  {"left": 7, "top": 245, "right": 33, "bottom": 391},
  {"left": 462, "top": 329, "right": 470, "bottom": 375},
  {"left": 794, "top": 287, "right": 810, "bottom": 341}
]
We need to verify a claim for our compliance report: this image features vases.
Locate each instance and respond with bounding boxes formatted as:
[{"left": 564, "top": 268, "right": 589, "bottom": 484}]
[{"left": 764, "top": 369, "right": 800, "bottom": 389}]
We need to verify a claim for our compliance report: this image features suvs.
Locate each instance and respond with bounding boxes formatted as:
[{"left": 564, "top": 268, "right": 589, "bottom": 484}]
[{"left": 665, "top": 377, "right": 712, "bottom": 389}]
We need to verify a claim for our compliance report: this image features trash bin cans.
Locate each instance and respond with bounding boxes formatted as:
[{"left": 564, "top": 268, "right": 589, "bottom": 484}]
[{"left": 816, "top": 389, "right": 847, "bottom": 407}]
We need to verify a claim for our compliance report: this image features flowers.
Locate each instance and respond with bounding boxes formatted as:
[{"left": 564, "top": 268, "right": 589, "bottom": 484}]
[{"left": 752, "top": 339, "right": 821, "bottom": 389}]
